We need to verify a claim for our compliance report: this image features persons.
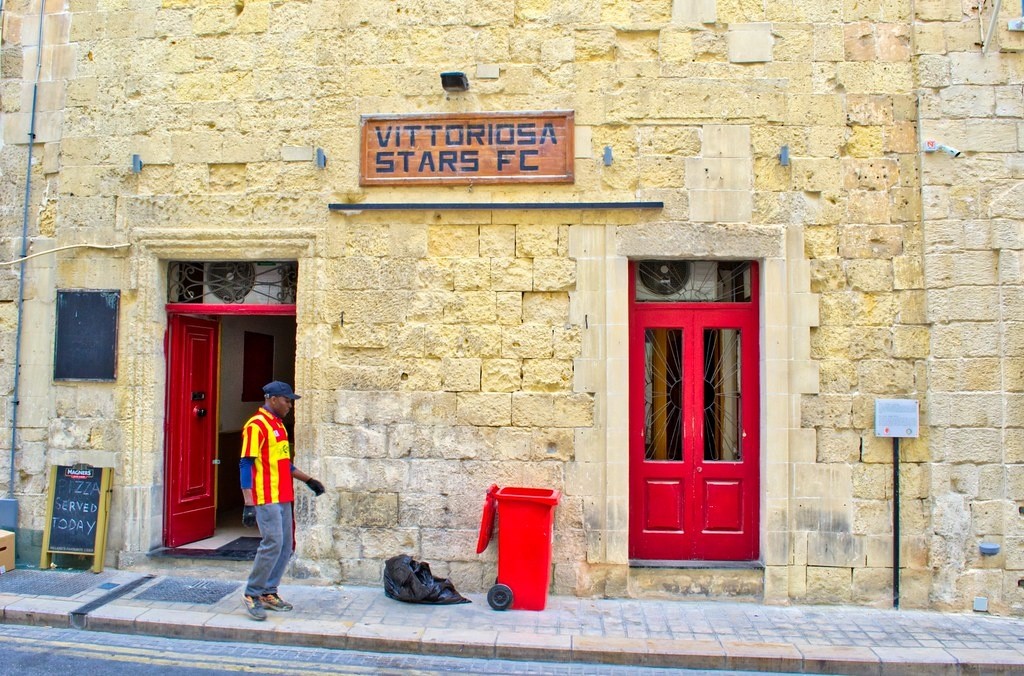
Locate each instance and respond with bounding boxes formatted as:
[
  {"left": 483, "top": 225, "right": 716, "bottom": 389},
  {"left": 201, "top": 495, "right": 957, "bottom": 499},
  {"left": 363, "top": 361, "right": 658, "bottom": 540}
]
[{"left": 239, "top": 381, "right": 325, "bottom": 619}]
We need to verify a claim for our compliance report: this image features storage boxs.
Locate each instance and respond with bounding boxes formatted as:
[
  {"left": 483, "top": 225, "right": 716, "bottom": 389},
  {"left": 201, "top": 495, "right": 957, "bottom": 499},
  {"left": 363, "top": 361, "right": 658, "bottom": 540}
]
[{"left": 0, "top": 529, "right": 15, "bottom": 575}]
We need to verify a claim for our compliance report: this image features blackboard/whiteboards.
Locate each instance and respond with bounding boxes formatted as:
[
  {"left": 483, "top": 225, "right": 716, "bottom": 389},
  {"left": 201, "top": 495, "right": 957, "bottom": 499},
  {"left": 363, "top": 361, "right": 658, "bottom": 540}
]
[
  {"left": 38, "top": 464, "right": 112, "bottom": 556},
  {"left": 51, "top": 288, "right": 121, "bottom": 384}
]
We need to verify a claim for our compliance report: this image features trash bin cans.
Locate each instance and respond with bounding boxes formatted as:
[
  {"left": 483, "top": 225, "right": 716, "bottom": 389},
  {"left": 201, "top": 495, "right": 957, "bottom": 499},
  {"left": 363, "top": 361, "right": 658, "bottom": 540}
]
[{"left": 475, "top": 482, "right": 561, "bottom": 613}]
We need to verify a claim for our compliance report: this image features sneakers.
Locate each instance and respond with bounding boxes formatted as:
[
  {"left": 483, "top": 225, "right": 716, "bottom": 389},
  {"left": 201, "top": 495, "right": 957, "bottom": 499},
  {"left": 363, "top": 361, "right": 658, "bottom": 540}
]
[
  {"left": 241, "top": 593, "right": 267, "bottom": 620},
  {"left": 261, "top": 593, "right": 293, "bottom": 612}
]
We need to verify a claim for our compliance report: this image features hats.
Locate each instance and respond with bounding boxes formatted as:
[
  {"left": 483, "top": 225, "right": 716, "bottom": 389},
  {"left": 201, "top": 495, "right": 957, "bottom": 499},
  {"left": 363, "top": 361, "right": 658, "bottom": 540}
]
[{"left": 262, "top": 380, "right": 302, "bottom": 399}]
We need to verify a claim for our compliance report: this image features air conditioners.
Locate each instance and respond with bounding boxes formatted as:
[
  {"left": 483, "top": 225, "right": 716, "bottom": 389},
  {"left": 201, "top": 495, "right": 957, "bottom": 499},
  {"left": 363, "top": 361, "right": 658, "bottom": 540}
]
[
  {"left": 202, "top": 262, "right": 293, "bottom": 304},
  {"left": 635, "top": 260, "right": 718, "bottom": 301}
]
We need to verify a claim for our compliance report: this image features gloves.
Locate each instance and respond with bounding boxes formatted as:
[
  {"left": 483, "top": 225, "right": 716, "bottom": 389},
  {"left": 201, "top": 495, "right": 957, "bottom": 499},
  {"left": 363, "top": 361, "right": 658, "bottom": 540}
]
[
  {"left": 242, "top": 504, "right": 256, "bottom": 528},
  {"left": 306, "top": 478, "right": 325, "bottom": 496}
]
